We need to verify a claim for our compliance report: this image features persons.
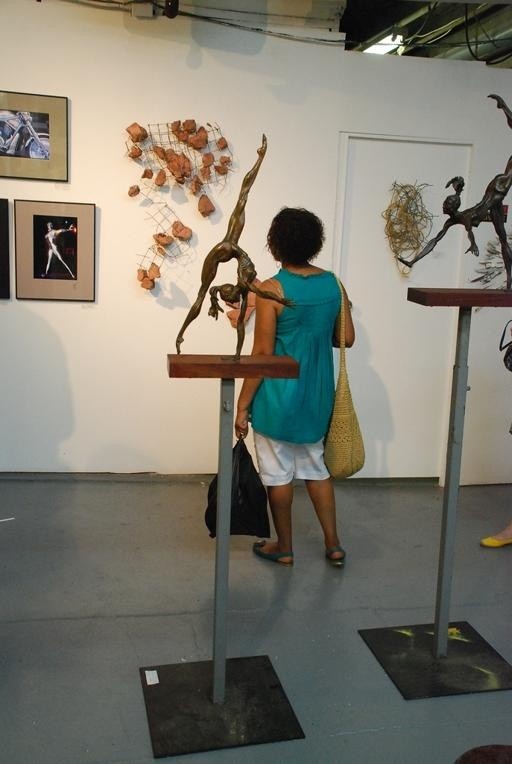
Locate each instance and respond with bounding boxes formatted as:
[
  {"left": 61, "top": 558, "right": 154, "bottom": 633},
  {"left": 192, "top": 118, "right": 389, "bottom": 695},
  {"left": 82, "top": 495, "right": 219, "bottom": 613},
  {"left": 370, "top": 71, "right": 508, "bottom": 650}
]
[
  {"left": 42, "top": 222, "right": 75, "bottom": 280},
  {"left": 396, "top": 94, "right": 512, "bottom": 288},
  {"left": 235, "top": 207, "right": 355, "bottom": 569},
  {"left": 479, "top": 523, "right": 512, "bottom": 546},
  {"left": 175, "top": 134, "right": 296, "bottom": 358}
]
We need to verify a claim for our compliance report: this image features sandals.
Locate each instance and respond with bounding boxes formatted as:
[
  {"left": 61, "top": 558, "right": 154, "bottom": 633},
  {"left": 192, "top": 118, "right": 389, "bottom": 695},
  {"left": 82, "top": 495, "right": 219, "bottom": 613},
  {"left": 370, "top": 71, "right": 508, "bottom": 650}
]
[
  {"left": 476, "top": 533, "right": 512, "bottom": 549},
  {"left": 252, "top": 540, "right": 295, "bottom": 568},
  {"left": 325, "top": 547, "right": 347, "bottom": 567}
]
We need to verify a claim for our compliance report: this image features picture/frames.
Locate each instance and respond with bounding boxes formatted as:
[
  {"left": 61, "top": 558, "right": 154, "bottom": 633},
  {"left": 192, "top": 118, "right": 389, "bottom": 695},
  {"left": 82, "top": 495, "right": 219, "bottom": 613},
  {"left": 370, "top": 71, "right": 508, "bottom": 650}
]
[
  {"left": 324, "top": 132, "right": 477, "bottom": 300},
  {"left": 0, "top": 87, "right": 70, "bottom": 182},
  {"left": 13, "top": 199, "right": 96, "bottom": 303}
]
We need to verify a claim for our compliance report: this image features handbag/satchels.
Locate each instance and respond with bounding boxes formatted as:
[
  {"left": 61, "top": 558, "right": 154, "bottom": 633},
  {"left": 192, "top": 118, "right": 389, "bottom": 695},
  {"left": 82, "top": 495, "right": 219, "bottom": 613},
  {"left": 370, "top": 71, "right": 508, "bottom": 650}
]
[{"left": 326, "top": 273, "right": 365, "bottom": 479}]
